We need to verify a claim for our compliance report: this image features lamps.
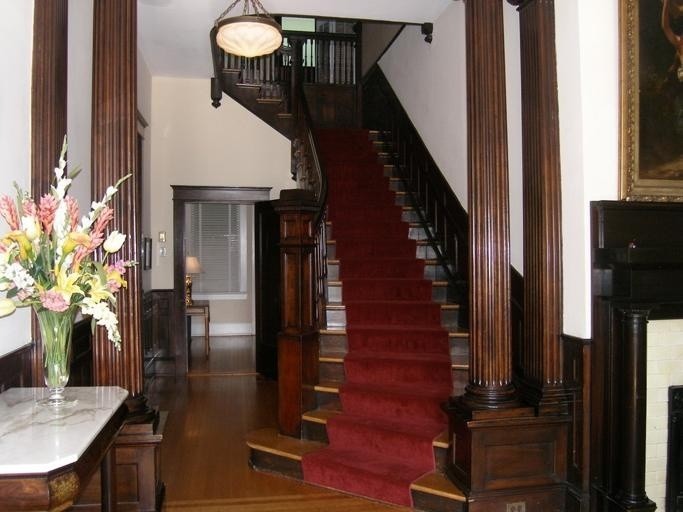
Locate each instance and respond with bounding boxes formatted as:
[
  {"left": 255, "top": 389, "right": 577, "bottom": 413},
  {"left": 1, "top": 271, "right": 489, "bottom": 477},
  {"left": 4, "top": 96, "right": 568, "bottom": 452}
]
[
  {"left": 184, "top": 256, "right": 205, "bottom": 306},
  {"left": 209, "top": 0, "right": 283, "bottom": 59}
]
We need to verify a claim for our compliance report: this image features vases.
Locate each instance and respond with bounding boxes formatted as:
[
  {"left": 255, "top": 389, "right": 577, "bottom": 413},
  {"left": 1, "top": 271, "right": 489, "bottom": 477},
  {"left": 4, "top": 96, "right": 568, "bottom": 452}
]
[{"left": 27, "top": 293, "right": 82, "bottom": 409}]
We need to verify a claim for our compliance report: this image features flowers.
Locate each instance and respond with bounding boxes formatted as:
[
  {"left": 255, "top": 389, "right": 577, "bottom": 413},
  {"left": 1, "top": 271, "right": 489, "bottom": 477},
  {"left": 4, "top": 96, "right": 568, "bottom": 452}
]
[{"left": 0, "top": 134, "right": 137, "bottom": 391}]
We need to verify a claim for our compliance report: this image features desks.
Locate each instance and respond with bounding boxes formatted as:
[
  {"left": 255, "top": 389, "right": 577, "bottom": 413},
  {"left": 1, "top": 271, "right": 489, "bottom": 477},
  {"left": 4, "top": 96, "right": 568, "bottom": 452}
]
[
  {"left": 183, "top": 300, "right": 210, "bottom": 368},
  {"left": 0, "top": 386, "right": 128, "bottom": 512}
]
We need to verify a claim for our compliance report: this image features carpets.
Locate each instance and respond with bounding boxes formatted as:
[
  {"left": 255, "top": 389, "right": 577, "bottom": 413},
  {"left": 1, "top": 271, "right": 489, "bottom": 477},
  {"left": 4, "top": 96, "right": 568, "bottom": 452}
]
[{"left": 301, "top": 127, "right": 455, "bottom": 510}]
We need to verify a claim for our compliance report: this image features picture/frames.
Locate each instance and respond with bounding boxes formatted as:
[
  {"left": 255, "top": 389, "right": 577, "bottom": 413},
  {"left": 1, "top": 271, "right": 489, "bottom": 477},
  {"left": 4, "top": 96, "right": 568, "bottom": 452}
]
[
  {"left": 142, "top": 238, "right": 152, "bottom": 271},
  {"left": 616, "top": 0, "right": 683, "bottom": 203}
]
